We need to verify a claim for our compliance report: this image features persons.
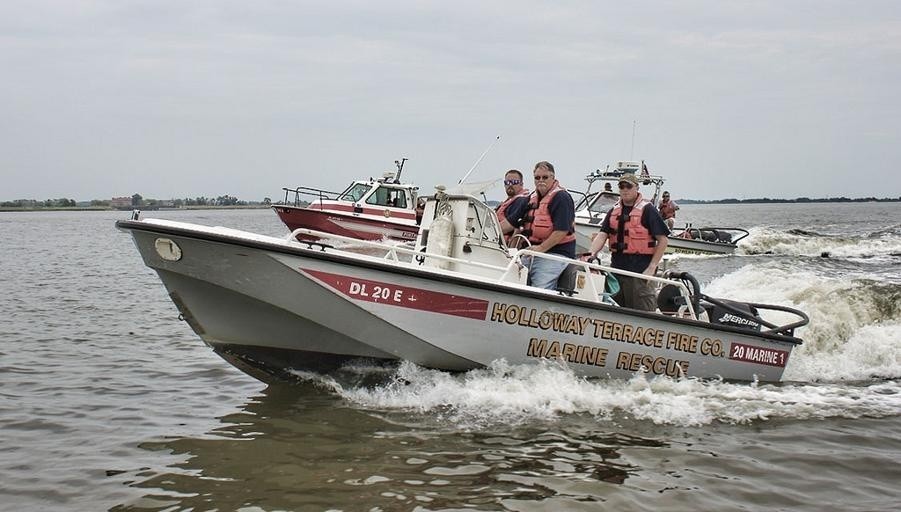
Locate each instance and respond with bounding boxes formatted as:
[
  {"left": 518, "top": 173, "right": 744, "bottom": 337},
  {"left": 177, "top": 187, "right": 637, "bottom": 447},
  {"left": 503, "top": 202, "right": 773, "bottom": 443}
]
[
  {"left": 495, "top": 168, "right": 532, "bottom": 245},
  {"left": 596, "top": 181, "right": 619, "bottom": 203},
  {"left": 581, "top": 173, "right": 672, "bottom": 313},
  {"left": 499, "top": 160, "right": 578, "bottom": 291},
  {"left": 658, "top": 191, "right": 680, "bottom": 232}
]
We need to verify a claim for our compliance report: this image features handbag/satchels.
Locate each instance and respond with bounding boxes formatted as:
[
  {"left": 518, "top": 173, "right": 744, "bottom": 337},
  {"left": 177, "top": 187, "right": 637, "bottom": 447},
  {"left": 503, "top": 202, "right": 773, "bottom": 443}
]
[{"left": 604, "top": 272, "right": 620, "bottom": 294}]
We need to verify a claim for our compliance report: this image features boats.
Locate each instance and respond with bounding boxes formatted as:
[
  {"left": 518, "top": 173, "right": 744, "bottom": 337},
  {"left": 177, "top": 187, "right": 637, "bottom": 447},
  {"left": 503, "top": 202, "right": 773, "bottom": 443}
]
[{"left": 106, "top": 134, "right": 811, "bottom": 385}]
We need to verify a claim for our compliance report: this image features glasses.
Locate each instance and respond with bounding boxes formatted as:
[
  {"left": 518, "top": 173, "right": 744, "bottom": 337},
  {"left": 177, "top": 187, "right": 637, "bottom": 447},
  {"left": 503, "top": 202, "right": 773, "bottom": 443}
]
[
  {"left": 535, "top": 176, "right": 548, "bottom": 179},
  {"left": 619, "top": 184, "right": 632, "bottom": 189},
  {"left": 504, "top": 180, "right": 521, "bottom": 185}
]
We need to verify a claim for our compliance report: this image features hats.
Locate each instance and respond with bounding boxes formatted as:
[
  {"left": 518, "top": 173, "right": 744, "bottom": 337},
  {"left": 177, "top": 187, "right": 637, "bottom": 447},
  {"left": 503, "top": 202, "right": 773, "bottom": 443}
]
[{"left": 619, "top": 175, "right": 638, "bottom": 185}]
